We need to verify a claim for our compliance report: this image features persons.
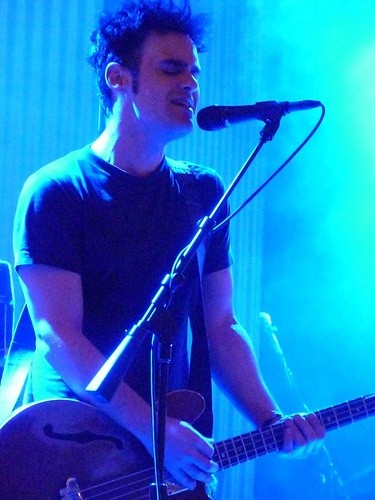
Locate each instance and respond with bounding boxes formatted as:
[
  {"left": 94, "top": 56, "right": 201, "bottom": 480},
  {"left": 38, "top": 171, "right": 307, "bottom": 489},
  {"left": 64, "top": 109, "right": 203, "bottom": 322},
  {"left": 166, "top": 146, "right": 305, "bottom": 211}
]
[{"left": 16, "top": 0, "right": 328, "bottom": 499}]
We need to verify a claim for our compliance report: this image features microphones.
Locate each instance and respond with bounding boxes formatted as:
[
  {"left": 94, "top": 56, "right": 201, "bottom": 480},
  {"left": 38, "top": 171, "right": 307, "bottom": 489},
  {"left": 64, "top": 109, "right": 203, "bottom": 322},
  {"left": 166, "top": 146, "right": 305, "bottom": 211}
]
[{"left": 196, "top": 99, "right": 321, "bottom": 132}]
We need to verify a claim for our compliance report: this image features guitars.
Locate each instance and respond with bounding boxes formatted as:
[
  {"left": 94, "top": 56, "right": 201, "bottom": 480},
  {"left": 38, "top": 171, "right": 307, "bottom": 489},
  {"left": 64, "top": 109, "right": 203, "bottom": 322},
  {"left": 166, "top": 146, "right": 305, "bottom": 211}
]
[{"left": 0, "top": 391, "right": 374, "bottom": 500}]
